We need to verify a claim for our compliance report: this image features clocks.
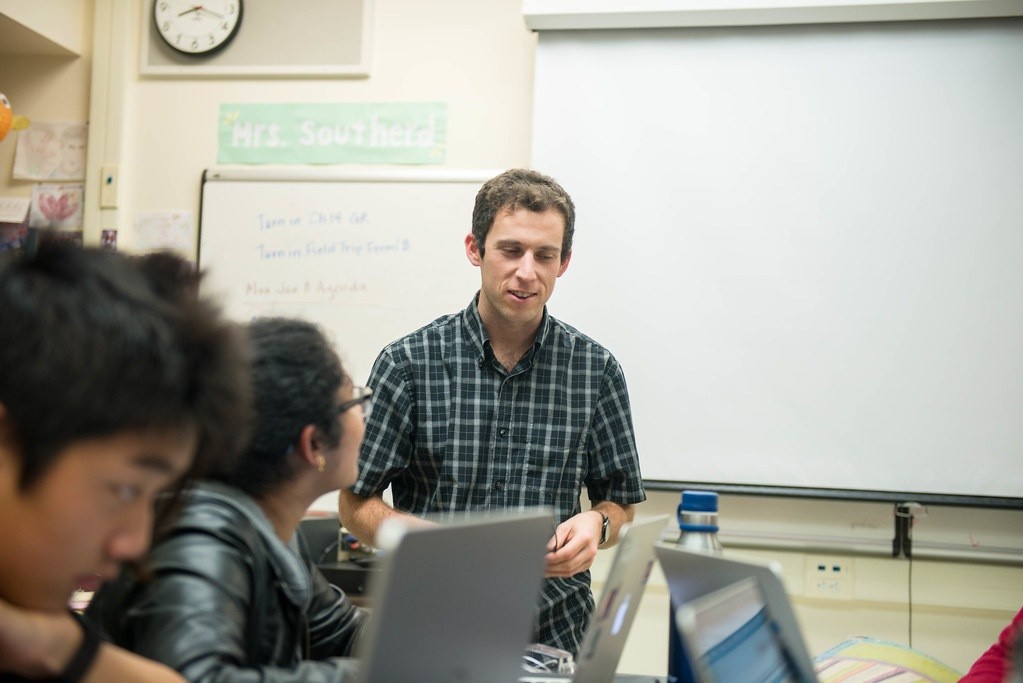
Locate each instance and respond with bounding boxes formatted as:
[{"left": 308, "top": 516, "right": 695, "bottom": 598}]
[{"left": 153, "top": 0, "right": 243, "bottom": 56}]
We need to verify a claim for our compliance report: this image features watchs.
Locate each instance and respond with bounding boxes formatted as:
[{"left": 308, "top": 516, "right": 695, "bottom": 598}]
[
  {"left": 596, "top": 509, "right": 611, "bottom": 547},
  {"left": 53, "top": 605, "right": 110, "bottom": 682}
]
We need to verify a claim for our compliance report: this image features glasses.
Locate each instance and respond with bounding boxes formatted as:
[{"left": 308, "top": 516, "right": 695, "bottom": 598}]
[{"left": 321, "top": 384, "right": 374, "bottom": 417}]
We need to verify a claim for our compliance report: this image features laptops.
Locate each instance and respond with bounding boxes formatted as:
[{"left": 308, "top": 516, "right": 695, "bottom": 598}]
[
  {"left": 523, "top": 513, "right": 670, "bottom": 683},
  {"left": 652, "top": 542, "right": 819, "bottom": 683},
  {"left": 358, "top": 504, "right": 554, "bottom": 683}
]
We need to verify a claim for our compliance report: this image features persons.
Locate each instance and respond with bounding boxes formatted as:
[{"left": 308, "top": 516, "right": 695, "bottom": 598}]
[
  {"left": 89, "top": 318, "right": 371, "bottom": 682},
  {"left": 336, "top": 168, "right": 648, "bottom": 675},
  {"left": 0, "top": 223, "right": 253, "bottom": 683}
]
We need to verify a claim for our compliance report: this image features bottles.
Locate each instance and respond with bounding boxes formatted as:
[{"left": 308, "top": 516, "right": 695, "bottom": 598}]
[{"left": 665, "top": 490, "right": 725, "bottom": 683}]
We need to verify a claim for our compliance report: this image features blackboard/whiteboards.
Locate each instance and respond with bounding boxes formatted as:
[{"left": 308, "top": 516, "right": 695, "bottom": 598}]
[{"left": 192, "top": 168, "right": 531, "bottom": 517}]
